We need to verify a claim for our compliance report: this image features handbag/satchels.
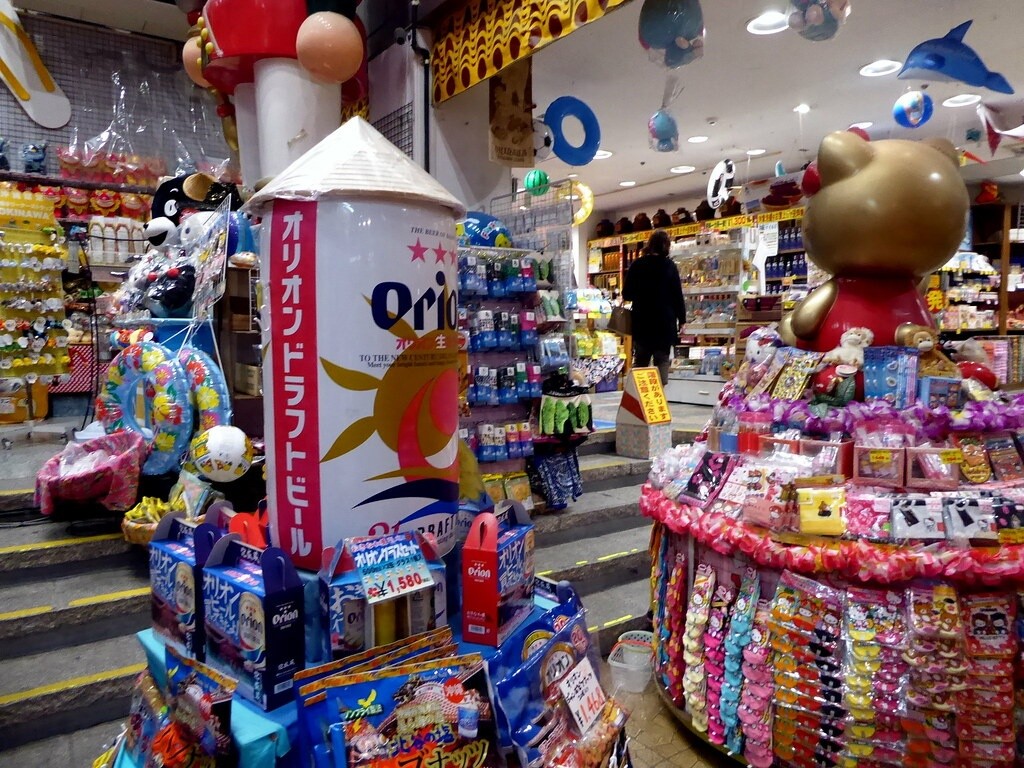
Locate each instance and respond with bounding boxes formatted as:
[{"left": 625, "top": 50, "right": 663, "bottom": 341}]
[{"left": 607, "top": 300, "right": 635, "bottom": 335}]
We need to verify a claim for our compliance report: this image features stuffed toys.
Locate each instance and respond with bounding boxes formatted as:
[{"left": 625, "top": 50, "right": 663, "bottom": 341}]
[{"left": 129, "top": 177, "right": 237, "bottom": 319}]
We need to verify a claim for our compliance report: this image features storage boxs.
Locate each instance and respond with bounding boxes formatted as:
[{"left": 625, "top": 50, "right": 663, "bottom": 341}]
[{"left": 0, "top": 143, "right": 1024, "bottom": 713}]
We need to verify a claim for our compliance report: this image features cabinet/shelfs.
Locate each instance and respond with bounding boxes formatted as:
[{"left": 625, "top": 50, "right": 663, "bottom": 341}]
[
  {"left": 224, "top": 264, "right": 259, "bottom": 335},
  {"left": 586, "top": 202, "right": 806, "bottom": 407},
  {"left": 939, "top": 200, "right": 1024, "bottom": 336},
  {"left": 490, "top": 197, "right": 587, "bottom": 289},
  {"left": 45, "top": 217, "right": 132, "bottom": 393},
  {"left": 460, "top": 247, "right": 550, "bottom": 516}
]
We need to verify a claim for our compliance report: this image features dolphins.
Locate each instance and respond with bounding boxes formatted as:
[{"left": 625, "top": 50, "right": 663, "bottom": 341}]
[{"left": 896, "top": 19, "right": 1015, "bottom": 95}]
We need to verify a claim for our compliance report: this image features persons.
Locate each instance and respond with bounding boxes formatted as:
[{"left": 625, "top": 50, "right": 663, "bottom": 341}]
[{"left": 622, "top": 232, "right": 688, "bottom": 386}]
